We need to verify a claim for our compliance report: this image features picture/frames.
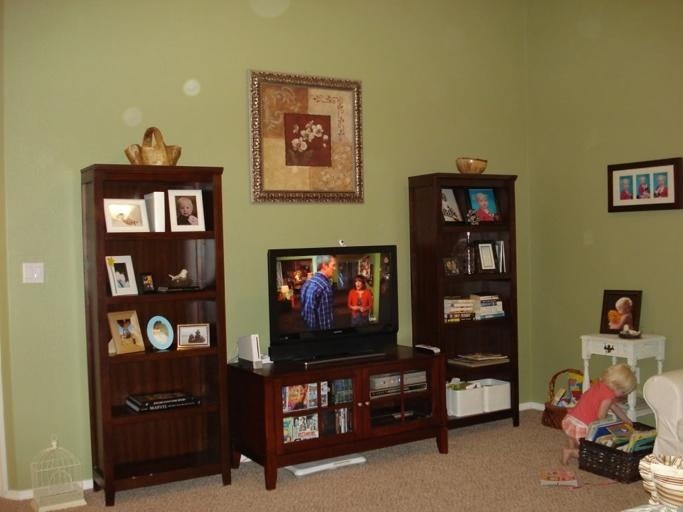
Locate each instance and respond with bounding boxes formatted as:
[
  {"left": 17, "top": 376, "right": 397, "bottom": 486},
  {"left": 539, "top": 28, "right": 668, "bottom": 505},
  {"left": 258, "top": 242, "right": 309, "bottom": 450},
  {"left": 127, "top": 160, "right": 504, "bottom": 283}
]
[
  {"left": 437, "top": 185, "right": 465, "bottom": 227},
  {"left": 604, "top": 154, "right": 683, "bottom": 214},
  {"left": 242, "top": 63, "right": 364, "bottom": 208},
  {"left": 95, "top": 181, "right": 215, "bottom": 360},
  {"left": 470, "top": 237, "right": 500, "bottom": 275},
  {"left": 597, "top": 284, "right": 643, "bottom": 337},
  {"left": 462, "top": 186, "right": 506, "bottom": 228}
]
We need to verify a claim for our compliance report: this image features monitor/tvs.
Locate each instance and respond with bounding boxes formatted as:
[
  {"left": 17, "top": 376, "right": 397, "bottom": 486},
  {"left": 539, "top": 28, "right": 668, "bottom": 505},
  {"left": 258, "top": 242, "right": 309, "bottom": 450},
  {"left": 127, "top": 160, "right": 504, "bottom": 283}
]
[{"left": 267, "top": 245, "right": 398, "bottom": 364}]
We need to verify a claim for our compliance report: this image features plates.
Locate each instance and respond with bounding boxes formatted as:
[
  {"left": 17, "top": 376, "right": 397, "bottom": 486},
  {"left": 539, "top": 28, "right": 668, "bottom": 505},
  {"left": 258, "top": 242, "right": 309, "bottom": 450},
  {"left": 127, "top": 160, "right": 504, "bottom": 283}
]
[{"left": 145, "top": 316, "right": 174, "bottom": 350}]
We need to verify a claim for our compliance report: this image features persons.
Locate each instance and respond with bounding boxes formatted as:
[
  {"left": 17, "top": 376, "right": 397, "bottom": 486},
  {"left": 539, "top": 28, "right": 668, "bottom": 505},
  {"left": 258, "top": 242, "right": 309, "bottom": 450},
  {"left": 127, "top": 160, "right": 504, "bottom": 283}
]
[
  {"left": 176, "top": 196, "right": 198, "bottom": 226},
  {"left": 300, "top": 255, "right": 337, "bottom": 331},
  {"left": 114, "top": 264, "right": 130, "bottom": 289},
  {"left": 441, "top": 193, "right": 460, "bottom": 223},
  {"left": 620, "top": 173, "right": 667, "bottom": 201},
  {"left": 117, "top": 319, "right": 136, "bottom": 345},
  {"left": 347, "top": 274, "right": 373, "bottom": 326},
  {"left": 607, "top": 296, "right": 633, "bottom": 330},
  {"left": 291, "top": 270, "right": 304, "bottom": 320},
  {"left": 562, "top": 363, "right": 640, "bottom": 463},
  {"left": 473, "top": 192, "right": 496, "bottom": 222}
]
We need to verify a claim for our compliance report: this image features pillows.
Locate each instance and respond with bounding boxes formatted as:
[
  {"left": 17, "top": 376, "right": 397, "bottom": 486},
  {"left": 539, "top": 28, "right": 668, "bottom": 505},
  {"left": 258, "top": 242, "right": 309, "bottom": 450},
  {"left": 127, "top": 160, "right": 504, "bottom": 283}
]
[{"left": 632, "top": 449, "right": 683, "bottom": 508}]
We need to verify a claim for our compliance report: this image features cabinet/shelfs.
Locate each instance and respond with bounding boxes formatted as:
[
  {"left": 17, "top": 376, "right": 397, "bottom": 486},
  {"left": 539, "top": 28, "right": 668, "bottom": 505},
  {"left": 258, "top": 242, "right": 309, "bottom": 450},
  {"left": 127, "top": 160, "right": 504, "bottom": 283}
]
[
  {"left": 220, "top": 339, "right": 452, "bottom": 494},
  {"left": 71, "top": 157, "right": 238, "bottom": 508},
  {"left": 406, "top": 166, "right": 524, "bottom": 432}
]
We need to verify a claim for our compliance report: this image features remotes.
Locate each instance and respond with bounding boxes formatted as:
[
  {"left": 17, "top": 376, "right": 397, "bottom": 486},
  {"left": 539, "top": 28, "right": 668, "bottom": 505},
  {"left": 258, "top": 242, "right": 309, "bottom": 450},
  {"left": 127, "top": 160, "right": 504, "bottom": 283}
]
[{"left": 413, "top": 343, "right": 441, "bottom": 355}]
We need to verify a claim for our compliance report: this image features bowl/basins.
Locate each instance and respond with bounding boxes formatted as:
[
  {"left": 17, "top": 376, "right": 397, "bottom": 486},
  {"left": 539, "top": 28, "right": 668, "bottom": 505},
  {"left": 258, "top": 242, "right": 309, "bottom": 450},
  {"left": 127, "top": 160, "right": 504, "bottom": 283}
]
[{"left": 454, "top": 157, "right": 487, "bottom": 174}]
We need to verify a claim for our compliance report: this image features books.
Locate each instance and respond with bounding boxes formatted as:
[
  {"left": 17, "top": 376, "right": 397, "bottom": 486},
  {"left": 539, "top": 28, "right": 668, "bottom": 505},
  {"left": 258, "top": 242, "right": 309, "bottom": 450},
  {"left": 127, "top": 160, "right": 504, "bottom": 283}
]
[
  {"left": 538, "top": 471, "right": 577, "bottom": 486},
  {"left": 443, "top": 239, "right": 512, "bottom": 421},
  {"left": 583, "top": 417, "right": 659, "bottom": 483},
  {"left": 124, "top": 391, "right": 203, "bottom": 413},
  {"left": 281, "top": 375, "right": 354, "bottom": 446}
]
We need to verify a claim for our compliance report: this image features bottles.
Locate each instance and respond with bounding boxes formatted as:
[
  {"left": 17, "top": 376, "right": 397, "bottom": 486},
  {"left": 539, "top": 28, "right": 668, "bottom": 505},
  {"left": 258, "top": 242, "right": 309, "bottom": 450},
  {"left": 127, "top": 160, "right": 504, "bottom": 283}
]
[{"left": 463, "top": 232, "right": 475, "bottom": 274}]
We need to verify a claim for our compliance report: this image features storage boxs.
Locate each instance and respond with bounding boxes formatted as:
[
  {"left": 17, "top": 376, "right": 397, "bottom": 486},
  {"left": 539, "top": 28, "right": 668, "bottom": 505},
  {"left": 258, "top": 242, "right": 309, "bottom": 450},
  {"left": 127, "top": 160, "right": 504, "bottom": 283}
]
[{"left": 445, "top": 375, "right": 512, "bottom": 419}]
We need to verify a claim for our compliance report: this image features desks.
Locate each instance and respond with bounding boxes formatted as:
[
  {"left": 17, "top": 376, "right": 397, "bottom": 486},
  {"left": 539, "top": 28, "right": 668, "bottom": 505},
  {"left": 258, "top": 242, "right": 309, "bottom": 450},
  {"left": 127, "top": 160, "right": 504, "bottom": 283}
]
[{"left": 576, "top": 330, "right": 670, "bottom": 426}]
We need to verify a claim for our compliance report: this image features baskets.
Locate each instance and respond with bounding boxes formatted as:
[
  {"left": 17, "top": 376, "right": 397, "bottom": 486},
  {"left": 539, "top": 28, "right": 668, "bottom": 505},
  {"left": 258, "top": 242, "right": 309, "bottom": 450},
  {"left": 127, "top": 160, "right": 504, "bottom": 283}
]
[
  {"left": 540, "top": 368, "right": 584, "bottom": 430},
  {"left": 578, "top": 421, "right": 657, "bottom": 484},
  {"left": 124, "top": 127, "right": 180, "bottom": 166}
]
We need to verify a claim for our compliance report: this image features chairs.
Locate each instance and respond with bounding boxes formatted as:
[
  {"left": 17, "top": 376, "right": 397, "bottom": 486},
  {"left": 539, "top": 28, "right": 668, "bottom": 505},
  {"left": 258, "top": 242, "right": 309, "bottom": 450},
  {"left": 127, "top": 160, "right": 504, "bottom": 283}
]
[{"left": 611, "top": 364, "right": 683, "bottom": 512}]
